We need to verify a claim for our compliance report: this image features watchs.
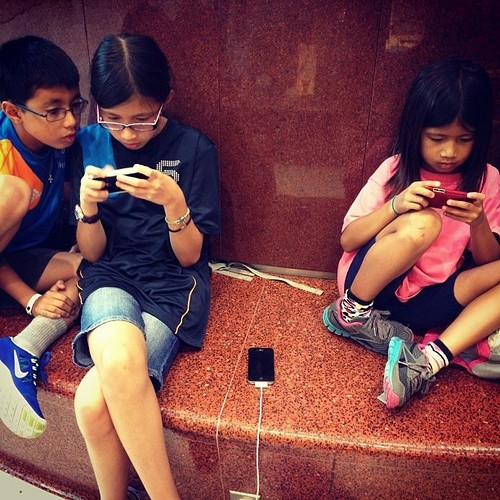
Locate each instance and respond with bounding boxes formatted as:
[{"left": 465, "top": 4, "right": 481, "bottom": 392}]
[{"left": 74, "top": 202, "right": 101, "bottom": 224}]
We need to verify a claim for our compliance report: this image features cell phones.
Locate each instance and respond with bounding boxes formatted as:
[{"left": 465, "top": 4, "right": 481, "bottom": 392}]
[
  {"left": 247, "top": 347, "right": 274, "bottom": 385},
  {"left": 416, "top": 186, "right": 473, "bottom": 212},
  {"left": 92, "top": 165, "right": 147, "bottom": 195}
]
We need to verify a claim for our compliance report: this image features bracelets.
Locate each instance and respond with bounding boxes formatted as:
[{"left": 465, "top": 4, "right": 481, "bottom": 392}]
[
  {"left": 25, "top": 293, "right": 42, "bottom": 316},
  {"left": 392, "top": 194, "right": 401, "bottom": 216},
  {"left": 166, "top": 216, "right": 191, "bottom": 233},
  {"left": 165, "top": 207, "right": 190, "bottom": 225}
]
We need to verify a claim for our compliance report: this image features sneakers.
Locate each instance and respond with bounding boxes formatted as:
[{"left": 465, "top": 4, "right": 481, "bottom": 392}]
[
  {"left": 0, "top": 336, "right": 52, "bottom": 439},
  {"left": 384, "top": 336, "right": 436, "bottom": 408},
  {"left": 322, "top": 296, "right": 414, "bottom": 355}
]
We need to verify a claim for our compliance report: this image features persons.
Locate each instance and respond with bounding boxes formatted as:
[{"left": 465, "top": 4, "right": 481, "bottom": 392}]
[
  {"left": 74, "top": 31, "right": 222, "bottom": 500},
  {"left": 0, "top": 35, "right": 88, "bottom": 439},
  {"left": 323, "top": 58, "right": 500, "bottom": 410}
]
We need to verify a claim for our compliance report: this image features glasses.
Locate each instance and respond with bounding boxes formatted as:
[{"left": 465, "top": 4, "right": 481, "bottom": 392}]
[
  {"left": 14, "top": 97, "right": 88, "bottom": 123},
  {"left": 96, "top": 103, "right": 162, "bottom": 131}
]
